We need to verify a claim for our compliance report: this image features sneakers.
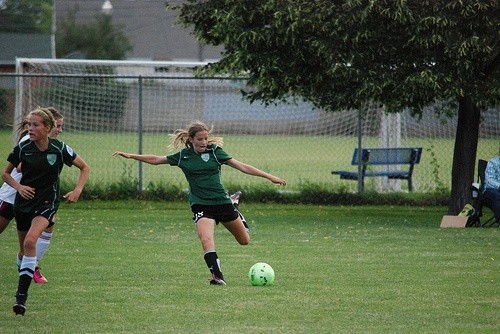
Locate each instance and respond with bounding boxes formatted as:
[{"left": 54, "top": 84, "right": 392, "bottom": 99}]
[
  {"left": 33, "top": 267, "right": 48, "bottom": 283},
  {"left": 230, "top": 191, "right": 242, "bottom": 204},
  {"left": 12, "top": 302, "right": 26, "bottom": 315},
  {"left": 208, "top": 273, "right": 226, "bottom": 285}
]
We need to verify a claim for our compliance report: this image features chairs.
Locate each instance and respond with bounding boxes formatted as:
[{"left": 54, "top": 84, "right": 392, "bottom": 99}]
[{"left": 470, "top": 158, "right": 497, "bottom": 227}]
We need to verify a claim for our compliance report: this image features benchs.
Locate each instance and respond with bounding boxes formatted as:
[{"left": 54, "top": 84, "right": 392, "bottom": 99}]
[{"left": 331, "top": 146, "right": 423, "bottom": 193}]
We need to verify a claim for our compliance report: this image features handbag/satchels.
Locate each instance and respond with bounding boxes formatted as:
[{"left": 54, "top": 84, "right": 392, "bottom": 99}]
[{"left": 458, "top": 203, "right": 496, "bottom": 226}]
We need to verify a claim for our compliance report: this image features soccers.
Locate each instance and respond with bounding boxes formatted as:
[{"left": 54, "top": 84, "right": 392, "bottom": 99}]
[{"left": 248, "top": 262, "right": 276, "bottom": 288}]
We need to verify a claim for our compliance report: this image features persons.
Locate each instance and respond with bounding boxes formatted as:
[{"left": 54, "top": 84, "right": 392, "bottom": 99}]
[
  {"left": 112, "top": 118, "right": 286, "bottom": 289},
  {"left": 482, "top": 145, "right": 500, "bottom": 227},
  {"left": 0, "top": 107, "right": 90, "bottom": 317}
]
